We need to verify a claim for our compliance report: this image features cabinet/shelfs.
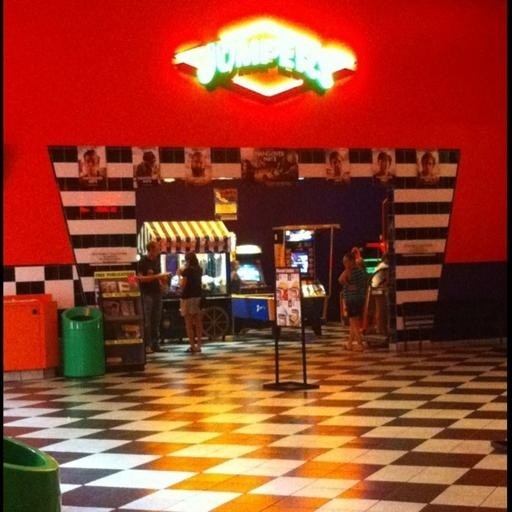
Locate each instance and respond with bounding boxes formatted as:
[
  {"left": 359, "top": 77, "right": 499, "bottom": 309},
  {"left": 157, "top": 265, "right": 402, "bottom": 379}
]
[{"left": 94, "top": 270, "right": 146, "bottom": 371}]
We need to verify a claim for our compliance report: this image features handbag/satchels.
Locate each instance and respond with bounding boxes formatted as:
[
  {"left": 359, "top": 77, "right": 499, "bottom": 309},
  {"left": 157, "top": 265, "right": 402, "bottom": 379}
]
[{"left": 174, "top": 285, "right": 183, "bottom": 297}]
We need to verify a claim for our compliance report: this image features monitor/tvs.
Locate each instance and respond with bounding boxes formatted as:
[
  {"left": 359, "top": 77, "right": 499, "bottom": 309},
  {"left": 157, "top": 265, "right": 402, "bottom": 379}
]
[
  {"left": 289, "top": 251, "right": 310, "bottom": 277},
  {"left": 362, "top": 256, "right": 381, "bottom": 276},
  {"left": 236, "top": 263, "right": 264, "bottom": 283}
]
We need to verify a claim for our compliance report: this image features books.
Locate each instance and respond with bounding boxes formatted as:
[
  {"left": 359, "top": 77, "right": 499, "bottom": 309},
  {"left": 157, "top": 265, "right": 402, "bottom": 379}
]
[{"left": 100, "top": 280, "right": 143, "bottom": 365}]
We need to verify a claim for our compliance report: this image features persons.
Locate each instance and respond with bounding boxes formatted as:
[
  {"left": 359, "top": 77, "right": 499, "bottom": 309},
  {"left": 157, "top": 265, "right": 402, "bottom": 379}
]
[
  {"left": 338, "top": 253, "right": 370, "bottom": 353},
  {"left": 263, "top": 151, "right": 299, "bottom": 182},
  {"left": 137, "top": 241, "right": 174, "bottom": 354},
  {"left": 372, "top": 152, "right": 396, "bottom": 182},
  {"left": 417, "top": 152, "right": 439, "bottom": 187},
  {"left": 241, "top": 159, "right": 257, "bottom": 183},
  {"left": 79, "top": 149, "right": 107, "bottom": 190},
  {"left": 175, "top": 251, "right": 206, "bottom": 353},
  {"left": 325, "top": 152, "right": 351, "bottom": 185},
  {"left": 189, "top": 153, "right": 208, "bottom": 178},
  {"left": 135, "top": 151, "right": 160, "bottom": 187},
  {"left": 369, "top": 252, "right": 390, "bottom": 335}
]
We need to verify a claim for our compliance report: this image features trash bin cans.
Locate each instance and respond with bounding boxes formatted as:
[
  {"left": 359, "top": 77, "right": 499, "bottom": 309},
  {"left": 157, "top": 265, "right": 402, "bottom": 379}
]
[
  {"left": 4, "top": 436, "right": 60, "bottom": 512},
  {"left": 62, "top": 307, "right": 106, "bottom": 378}
]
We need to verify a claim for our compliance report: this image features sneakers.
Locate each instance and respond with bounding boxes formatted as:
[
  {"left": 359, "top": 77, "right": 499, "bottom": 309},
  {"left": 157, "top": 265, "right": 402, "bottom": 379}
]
[
  {"left": 184, "top": 346, "right": 201, "bottom": 352},
  {"left": 145, "top": 344, "right": 168, "bottom": 354}
]
